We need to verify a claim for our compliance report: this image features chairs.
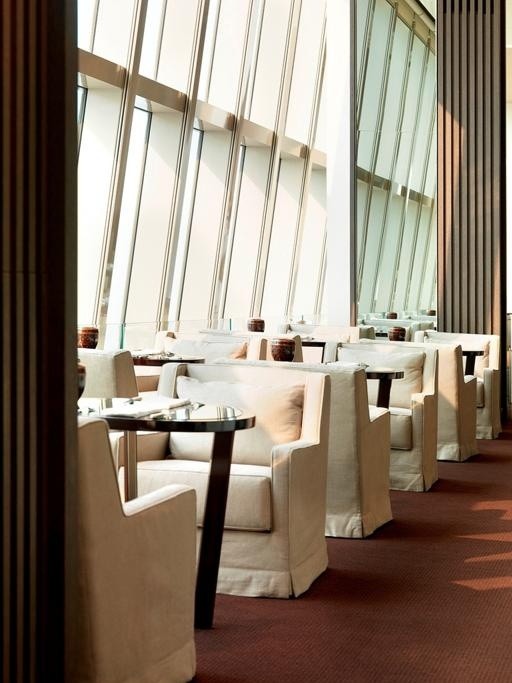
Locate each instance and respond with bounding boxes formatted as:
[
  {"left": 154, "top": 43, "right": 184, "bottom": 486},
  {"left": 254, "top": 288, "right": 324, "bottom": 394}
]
[{"left": 68, "top": 306, "right": 502, "bottom": 682}]
